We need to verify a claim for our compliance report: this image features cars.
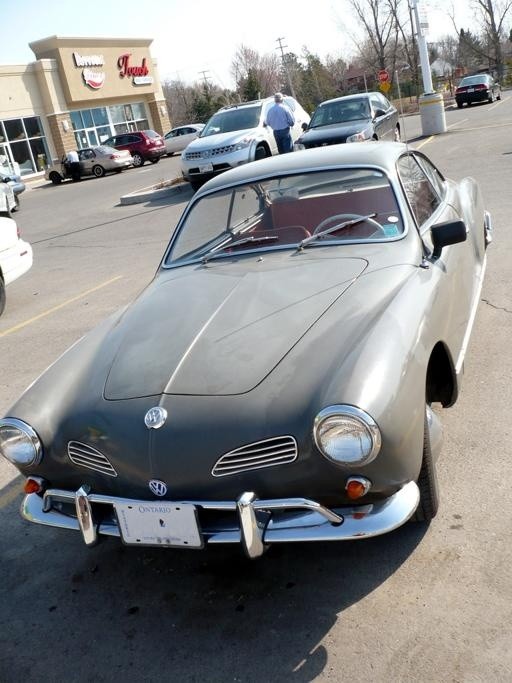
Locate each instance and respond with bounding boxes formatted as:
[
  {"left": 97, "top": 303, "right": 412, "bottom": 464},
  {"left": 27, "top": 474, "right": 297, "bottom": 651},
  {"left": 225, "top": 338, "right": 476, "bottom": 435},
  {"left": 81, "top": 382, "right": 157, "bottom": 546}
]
[
  {"left": 0, "top": 141, "right": 493, "bottom": 561},
  {"left": 293, "top": 92, "right": 401, "bottom": 152},
  {"left": 44, "top": 123, "right": 220, "bottom": 185},
  {"left": 0, "top": 172, "right": 33, "bottom": 317},
  {"left": 455, "top": 74, "right": 501, "bottom": 108},
  {"left": 180, "top": 94, "right": 311, "bottom": 192}
]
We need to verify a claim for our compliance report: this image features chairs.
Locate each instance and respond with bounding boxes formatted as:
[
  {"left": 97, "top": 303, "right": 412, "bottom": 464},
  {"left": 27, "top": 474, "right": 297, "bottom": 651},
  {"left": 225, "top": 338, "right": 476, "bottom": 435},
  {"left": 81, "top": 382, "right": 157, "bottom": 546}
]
[{"left": 229, "top": 178, "right": 433, "bottom": 249}]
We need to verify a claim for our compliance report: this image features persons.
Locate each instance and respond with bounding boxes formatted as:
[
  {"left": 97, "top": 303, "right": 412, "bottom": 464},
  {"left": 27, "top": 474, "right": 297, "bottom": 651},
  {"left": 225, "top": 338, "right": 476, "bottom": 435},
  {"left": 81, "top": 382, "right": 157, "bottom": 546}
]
[
  {"left": 266, "top": 93, "right": 295, "bottom": 154},
  {"left": 67, "top": 151, "right": 81, "bottom": 182}
]
